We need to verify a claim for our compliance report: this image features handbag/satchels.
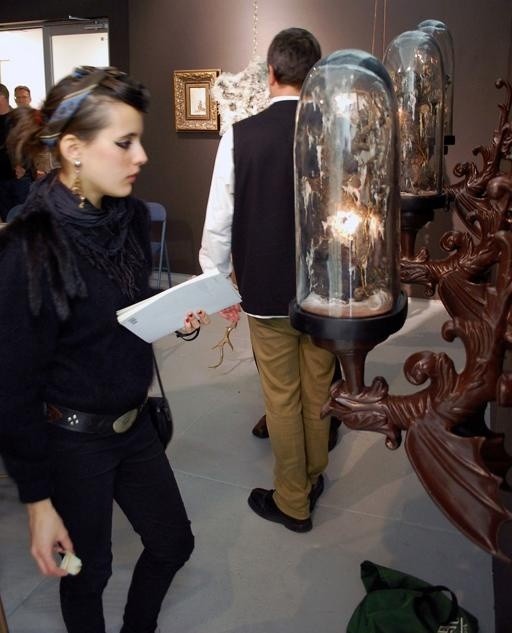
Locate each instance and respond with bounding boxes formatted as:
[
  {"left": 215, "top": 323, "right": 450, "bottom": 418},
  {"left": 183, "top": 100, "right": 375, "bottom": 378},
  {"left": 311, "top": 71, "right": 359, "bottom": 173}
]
[
  {"left": 346, "top": 560, "right": 479, "bottom": 633},
  {"left": 147, "top": 396, "right": 173, "bottom": 449}
]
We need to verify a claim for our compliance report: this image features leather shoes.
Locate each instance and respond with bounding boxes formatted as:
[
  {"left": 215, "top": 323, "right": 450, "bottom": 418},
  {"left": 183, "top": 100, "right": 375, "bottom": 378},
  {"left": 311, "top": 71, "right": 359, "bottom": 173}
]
[
  {"left": 328, "top": 415, "right": 342, "bottom": 452},
  {"left": 252, "top": 414, "right": 270, "bottom": 439},
  {"left": 248, "top": 474, "right": 325, "bottom": 533}
]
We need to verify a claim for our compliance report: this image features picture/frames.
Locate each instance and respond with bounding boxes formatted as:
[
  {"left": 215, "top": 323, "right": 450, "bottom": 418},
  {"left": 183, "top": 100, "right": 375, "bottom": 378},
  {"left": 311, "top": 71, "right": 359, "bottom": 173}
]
[{"left": 172, "top": 68, "right": 220, "bottom": 132}]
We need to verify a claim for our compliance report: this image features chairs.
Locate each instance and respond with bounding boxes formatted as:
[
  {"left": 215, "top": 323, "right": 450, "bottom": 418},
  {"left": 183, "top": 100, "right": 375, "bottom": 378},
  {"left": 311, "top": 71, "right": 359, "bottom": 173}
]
[{"left": 138, "top": 200, "right": 172, "bottom": 291}]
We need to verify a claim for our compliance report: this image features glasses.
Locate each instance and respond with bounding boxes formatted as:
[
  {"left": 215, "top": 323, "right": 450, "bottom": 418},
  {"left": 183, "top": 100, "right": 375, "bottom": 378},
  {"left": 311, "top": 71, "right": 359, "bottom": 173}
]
[{"left": 14, "top": 96, "right": 29, "bottom": 100}]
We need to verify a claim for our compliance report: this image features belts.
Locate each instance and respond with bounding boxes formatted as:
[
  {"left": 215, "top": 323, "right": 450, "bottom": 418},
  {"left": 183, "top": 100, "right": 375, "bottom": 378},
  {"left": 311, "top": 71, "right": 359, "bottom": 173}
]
[{"left": 41, "top": 401, "right": 148, "bottom": 438}]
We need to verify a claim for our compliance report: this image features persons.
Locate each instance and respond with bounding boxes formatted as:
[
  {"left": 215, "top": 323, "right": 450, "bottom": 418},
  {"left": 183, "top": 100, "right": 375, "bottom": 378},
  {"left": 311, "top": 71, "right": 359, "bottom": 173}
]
[
  {"left": 198, "top": 28, "right": 343, "bottom": 532},
  {"left": 0, "top": 84, "right": 38, "bottom": 219},
  {"left": 0, "top": 64, "right": 212, "bottom": 632}
]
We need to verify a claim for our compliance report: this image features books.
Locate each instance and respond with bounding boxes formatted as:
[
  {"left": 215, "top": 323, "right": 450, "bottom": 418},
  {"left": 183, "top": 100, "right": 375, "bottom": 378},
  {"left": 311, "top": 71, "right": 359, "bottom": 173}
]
[{"left": 115, "top": 267, "right": 243, "bottom": 344}]
